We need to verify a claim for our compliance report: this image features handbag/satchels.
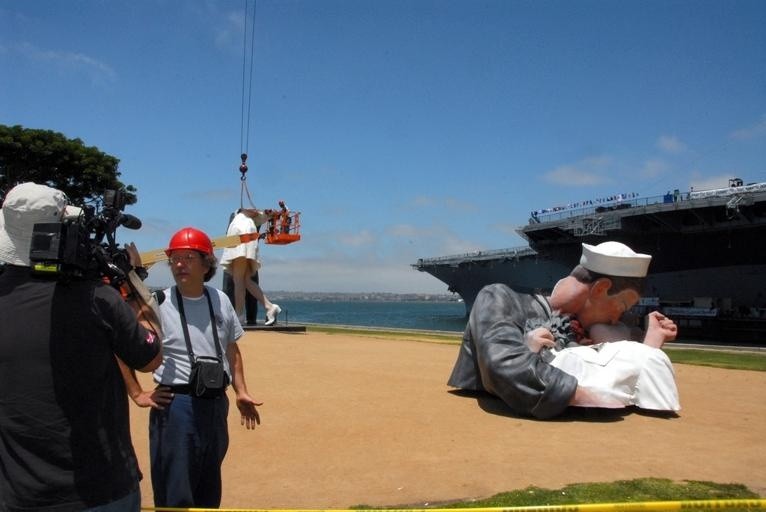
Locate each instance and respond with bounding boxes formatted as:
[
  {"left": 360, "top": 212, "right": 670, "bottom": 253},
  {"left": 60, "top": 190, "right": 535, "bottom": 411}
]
[{"left": 189, "top": 355, "right": 225, "bottom": 397}]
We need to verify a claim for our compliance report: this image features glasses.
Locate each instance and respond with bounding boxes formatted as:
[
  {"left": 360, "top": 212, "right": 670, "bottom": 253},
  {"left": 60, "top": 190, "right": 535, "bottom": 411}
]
[{"left": 167, "top": 253, "right": 198, "bottom": 265}]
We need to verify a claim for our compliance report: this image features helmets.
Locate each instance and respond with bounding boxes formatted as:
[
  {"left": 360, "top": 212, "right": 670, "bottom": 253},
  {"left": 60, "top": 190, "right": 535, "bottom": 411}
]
[
  {"left": 164, "top": 227, "right": 213, "bottom": 258},
  {"left": 279, "top": 201, "right": 284, "bottom": 205}
]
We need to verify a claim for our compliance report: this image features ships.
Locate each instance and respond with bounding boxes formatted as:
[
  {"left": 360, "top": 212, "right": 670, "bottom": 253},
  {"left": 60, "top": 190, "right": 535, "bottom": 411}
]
[{"left": 409, "top": 180, "right": 766, "bottom": 343}]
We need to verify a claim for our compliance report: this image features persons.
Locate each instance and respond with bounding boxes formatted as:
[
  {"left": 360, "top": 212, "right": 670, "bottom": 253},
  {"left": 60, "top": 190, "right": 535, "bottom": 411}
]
[
  {"left": 278, "top": 200, "right": 291, "bottom": 234},
  {"left": 0, "top": 180, "right": 164, "bottom": 512},
  {"left": 525, "top": 313, "right": 683, "bottom": 415},
  {"left": 219, "top": 207, "right": 282, "bottom": 327},
  {"left": 114, "top": 226, "right": 264, "bottom": 509},
  {"left": 446, "top": 239, "right": 679, "bottom": 421}
]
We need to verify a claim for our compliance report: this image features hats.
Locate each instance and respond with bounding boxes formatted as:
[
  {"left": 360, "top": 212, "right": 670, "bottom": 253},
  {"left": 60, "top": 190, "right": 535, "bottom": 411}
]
[{"left": 0, "top": 181, "right": 83, "bottom": 267}]
